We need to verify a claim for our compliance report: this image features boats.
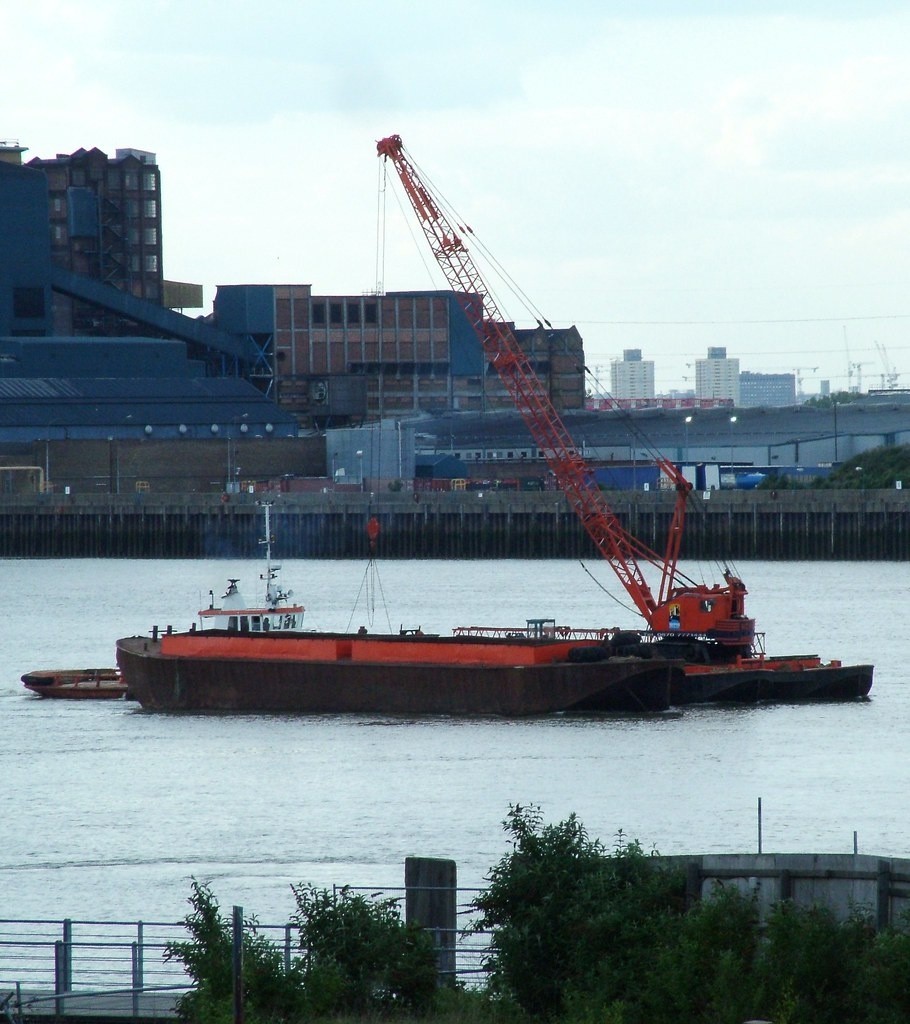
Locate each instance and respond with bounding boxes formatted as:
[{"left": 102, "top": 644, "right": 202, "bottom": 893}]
[{"left": 19, "top": 132, "right": 878, "bottom": 717}]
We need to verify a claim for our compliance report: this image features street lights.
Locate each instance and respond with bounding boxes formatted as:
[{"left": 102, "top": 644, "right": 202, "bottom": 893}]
[
  {"left": 683, "top": 414, "right": 694, "bottom": 465},
  {"left": 727, "top": 415, "right": 738, "bottom": 471},
  {"left": 112, "top": 415, "right": 135, "bottom": 492},
  {"left": 227, "top": 412, "right": 250, "bottom": 483}
]
